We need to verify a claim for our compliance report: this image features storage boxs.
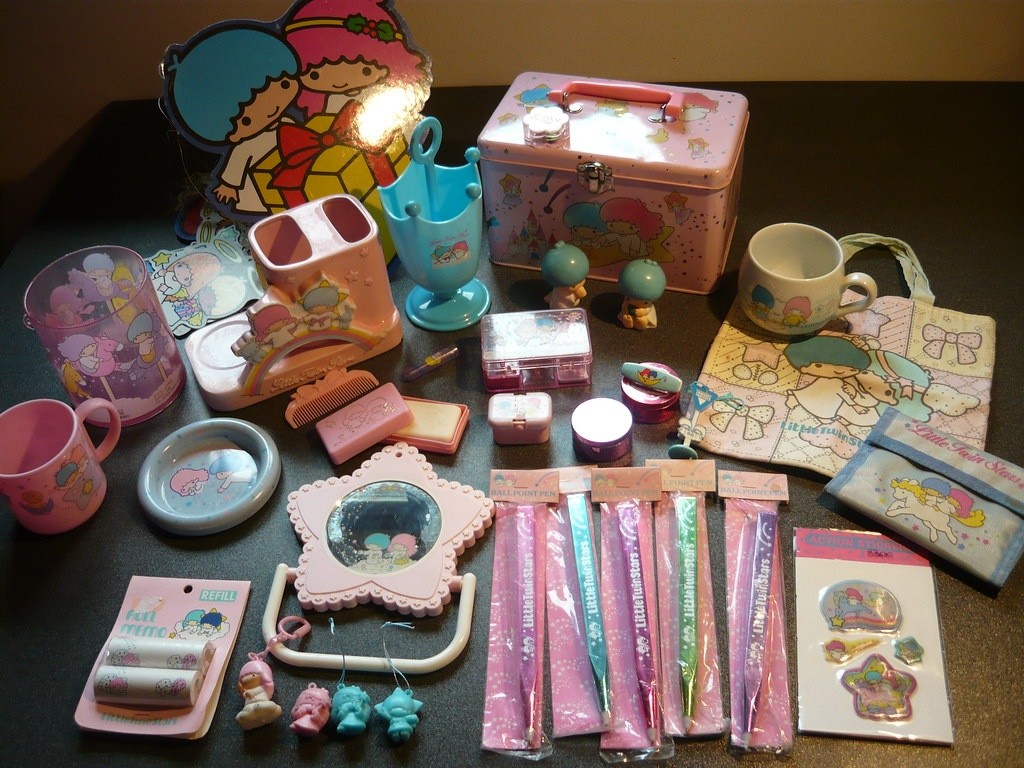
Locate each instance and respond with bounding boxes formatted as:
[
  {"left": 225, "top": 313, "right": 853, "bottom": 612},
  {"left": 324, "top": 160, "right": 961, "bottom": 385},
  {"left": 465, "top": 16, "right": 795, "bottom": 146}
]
[
  {"left": 315, "top": 382, "right": 470, "bottom": 467},
  {"left": 478, "top": 71, "right": 749, "bottom": 295},
  {"left": 480, "top": 308, "right": 593, "bottom": 445}
]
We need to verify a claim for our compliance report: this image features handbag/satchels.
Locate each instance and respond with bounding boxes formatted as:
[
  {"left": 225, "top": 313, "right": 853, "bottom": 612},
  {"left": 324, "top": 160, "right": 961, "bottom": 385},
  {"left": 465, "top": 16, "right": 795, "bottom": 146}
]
[{"left": 678, "top": 232, "right": 995, "bottom": 482}]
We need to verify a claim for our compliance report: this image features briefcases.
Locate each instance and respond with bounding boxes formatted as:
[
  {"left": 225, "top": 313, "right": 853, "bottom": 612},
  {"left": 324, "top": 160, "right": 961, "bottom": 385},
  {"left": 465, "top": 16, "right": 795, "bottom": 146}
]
[{"left": 476, "top": 70, "right": 750, "bottom": 297}]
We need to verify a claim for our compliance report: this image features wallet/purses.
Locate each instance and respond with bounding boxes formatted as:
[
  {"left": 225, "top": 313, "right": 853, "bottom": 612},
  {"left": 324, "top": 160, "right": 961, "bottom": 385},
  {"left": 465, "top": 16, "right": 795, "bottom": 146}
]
[{"left": 820, "top": 406, "right": 1024, "bottom": 598}]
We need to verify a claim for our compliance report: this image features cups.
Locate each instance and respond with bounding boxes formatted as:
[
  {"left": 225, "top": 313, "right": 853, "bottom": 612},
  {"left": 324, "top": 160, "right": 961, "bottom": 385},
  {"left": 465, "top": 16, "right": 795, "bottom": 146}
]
[
  {"left": 0, "top": 399, "right": 121, "bottom": 535},
  {"left": 23, "top": 246, "right": 186, "bottom": 428},
  {"left": 736, "top": 222, "right": 877, "bottom": 336}
]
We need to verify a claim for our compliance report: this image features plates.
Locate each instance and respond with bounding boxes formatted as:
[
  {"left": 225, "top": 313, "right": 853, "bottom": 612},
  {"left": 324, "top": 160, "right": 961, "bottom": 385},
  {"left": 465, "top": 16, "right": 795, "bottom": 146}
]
[{"left": 136, "top": 417, "right": 282, "bottom": 536}]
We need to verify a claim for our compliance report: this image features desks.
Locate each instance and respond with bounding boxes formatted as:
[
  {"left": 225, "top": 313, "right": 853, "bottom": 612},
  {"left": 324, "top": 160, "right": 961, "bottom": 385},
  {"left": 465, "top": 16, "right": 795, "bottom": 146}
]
[{"left": 0, "top": 79, "right": 1023, "bottom": 768}]
